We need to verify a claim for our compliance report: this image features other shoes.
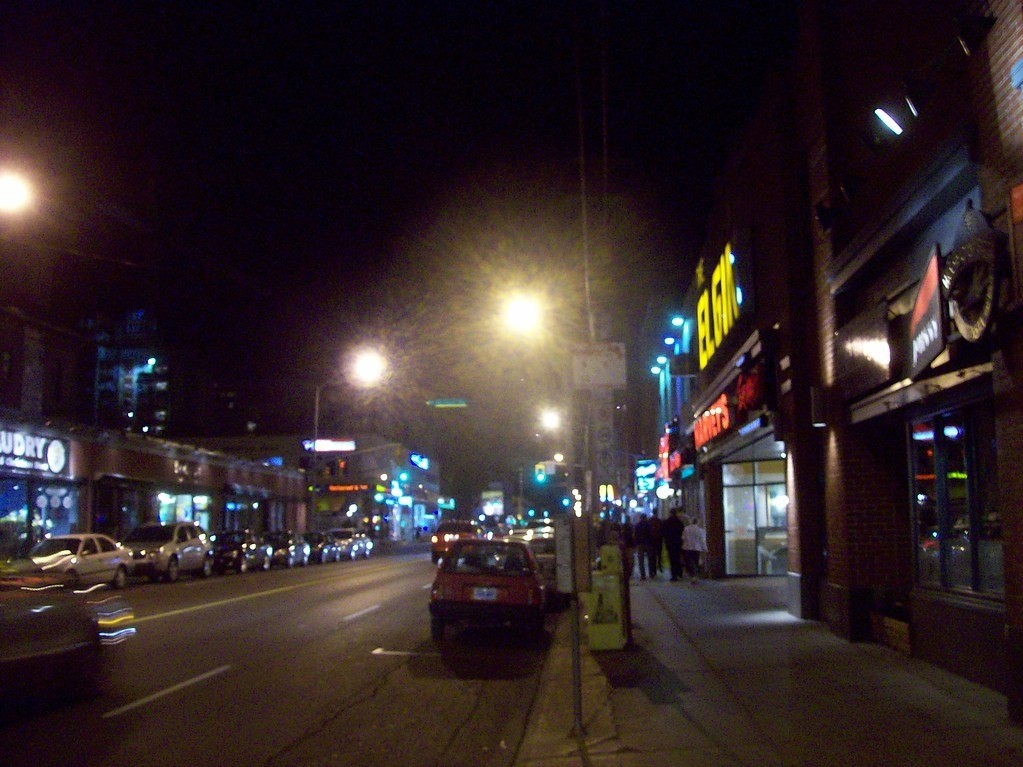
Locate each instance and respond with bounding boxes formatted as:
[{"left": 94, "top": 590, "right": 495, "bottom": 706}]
[
  {"left": 678, "top": 571, "right": 682, "bottom": 578},
  {"left": 649, "top": 572, "right": 655, "bottom": 578},
  {"left": 691, "top": 577, "right": 697, "bottom": 582},
  {"left": 670, "top": 575, "right": 677, "bottom": 581},
  {"left": 640, "top": 576, "right": 646, "bottom": 581}
]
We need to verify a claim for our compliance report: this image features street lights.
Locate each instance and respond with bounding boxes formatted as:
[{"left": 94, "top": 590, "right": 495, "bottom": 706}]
[
  {"left": 500, "top": 293, "right": 598, "bottom": 514},
  {"left": 554, "top": 454, "right": 576, "bottom": 517},
  {"left": 309, "top": 355, "right": 379, "bottom": 530},
  {"left": 539, "top": 408, "right": 590, "bottom": 511}
]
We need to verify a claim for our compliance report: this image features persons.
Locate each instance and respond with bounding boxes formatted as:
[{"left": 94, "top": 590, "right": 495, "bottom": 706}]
[
  {"left": 682, "top": 517, "right": 709, "bottom": 584},
  {"left": 596, "top": 502, "right": 692, "bottom": 583}
]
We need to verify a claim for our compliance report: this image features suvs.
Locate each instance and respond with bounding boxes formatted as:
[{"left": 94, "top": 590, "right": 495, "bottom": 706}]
[
  {"left": 432, "top": 519, "right": 485, "bottom": 563},
  {"left": 119, "top": 521, "right": 217, "bottom": 582}
]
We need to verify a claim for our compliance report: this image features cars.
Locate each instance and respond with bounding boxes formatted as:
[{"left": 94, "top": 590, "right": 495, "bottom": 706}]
[
  {"left": 304, "top": 530, "right": 341, "bottom": 564},
  {"left": 504, "top": 526, "right": 558, "bottom": 578},
  {"left": 332, "top": 529, "right": 374, "bottom": 561},
  {"left": 260, "top": 529, "right": 312, "bottom": 568},
  {"left": 0, "top": 574, "right": 115, "bottom": 724},
  {"left": 206, "top": 529, "right": 274, "bottom": 575},
  {"left": 27, "top": 533, "right": 135, "bottom": 591},
  {"left": 428, "top": 538, "right": 548, "bottom": 645},
  {"left": 530, "top": 518, "right": 554, "bottom": 540}
]
